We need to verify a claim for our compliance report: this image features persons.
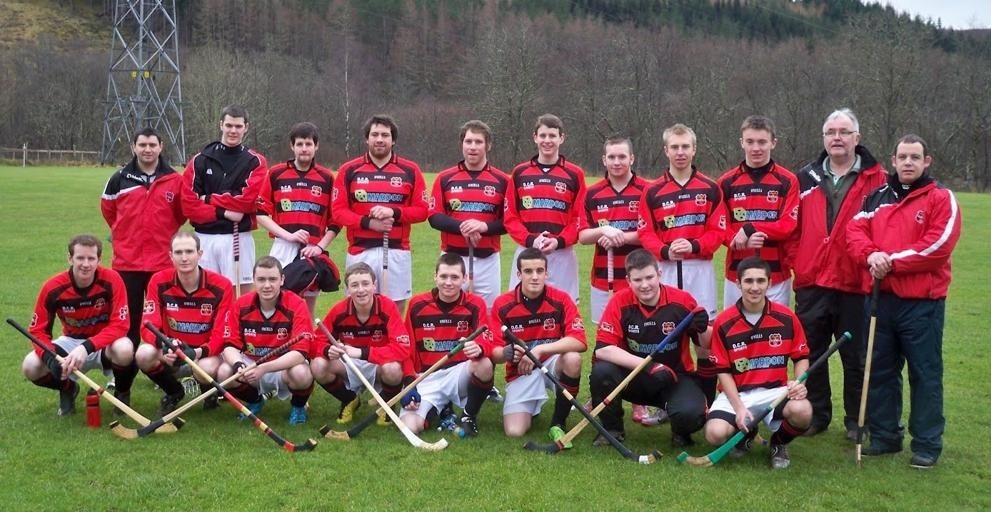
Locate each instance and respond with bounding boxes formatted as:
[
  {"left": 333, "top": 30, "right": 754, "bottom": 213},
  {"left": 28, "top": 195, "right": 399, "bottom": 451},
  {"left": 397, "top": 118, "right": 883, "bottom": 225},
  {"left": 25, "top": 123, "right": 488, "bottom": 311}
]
[
  {"left": 22, "top": 235, "right": 138, "bottom": 417},
  {"left": 428, "top": 113, "right": 812, "bottom": 425},
  {"left": 488, "top": 248, "right": 587, "bottom": 449},
  {"left": 134, "top": 232, "right": 234, "bottom": 411},
  {"left": 781, "top": 108, "right": 887, "bottom": 437},
  {"left": 845, "top": 132, "right": 964, "bottom": 473},
  {"left": 706, "top": 257, "right": 811, "bottom": 468},
  {"left": 256, "top": 122, "right": 343, "bottom": 313},
  {"left": 399, "top": 253, "right": 492, "bottom": 437},
  {"left": 100, "top": 127, "right": 187, "bottom": 391},
  {"left": 331, "top": 114, "right": 429, "bottom": 321},
  {"left": 181, "top": 103, "right": 267, "bottom": 299},
  {"left": 310, "top": 263, "right": 412, "bottom": 427},
  {"left": 215, "top": 255, "right": 313, "bottom": 425},
  {"left": 589, "top": 248, "right": 712, "bottom": 448}
]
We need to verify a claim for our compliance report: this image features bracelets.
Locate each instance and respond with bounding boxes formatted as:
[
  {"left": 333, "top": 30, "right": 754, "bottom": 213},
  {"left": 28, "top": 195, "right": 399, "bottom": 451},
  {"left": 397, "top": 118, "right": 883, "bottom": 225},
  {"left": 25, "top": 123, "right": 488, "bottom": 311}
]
[{"left": 316, "top": 244, "right": 324, "bottom": 253}]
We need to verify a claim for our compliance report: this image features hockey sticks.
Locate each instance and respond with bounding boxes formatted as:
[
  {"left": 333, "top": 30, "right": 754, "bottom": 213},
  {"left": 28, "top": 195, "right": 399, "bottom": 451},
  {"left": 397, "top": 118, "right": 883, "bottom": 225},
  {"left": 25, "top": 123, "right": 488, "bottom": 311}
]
[
  {"left": 369, "top": 229, "right": 389, "bottom": 407},
  {"left": 317, "top": 323, "right": 488, "bottom": 441},
  {"left": 675, "top": 329, "right": 854, "bottom": 469},
  {"left": 313, "top": 316, "right": 449, "bottom": 454},
  {"left": 143, "top": 320, "right": 319, "bottom": 454},
  {"left": 520, "top": 301, "right": 707, "bottom": 454},
  {"left": 5, "top": 316, "right": 186, "bottom": 435},
  {"left": 108, "top": 332, "right": 312, "bottom": 441},
  {"left": 754, "top": 247, "right": 770, "bottom": 448},
  {"left": 853, "top": 273, "right": 882, "bottom": 472},
  {"left": 499, "top": 324, "right": 664, "bottom": 467}
]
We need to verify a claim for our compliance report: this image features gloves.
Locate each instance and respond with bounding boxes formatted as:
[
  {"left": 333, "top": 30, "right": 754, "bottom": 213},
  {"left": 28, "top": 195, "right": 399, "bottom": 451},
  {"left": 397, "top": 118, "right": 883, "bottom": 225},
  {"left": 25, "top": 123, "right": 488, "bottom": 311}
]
[
  {"left": 687, "top": 307, "right": 709, "bottom": 335},
  {"left": 644, "top": 362, "right": 680, "bottom": 389}
]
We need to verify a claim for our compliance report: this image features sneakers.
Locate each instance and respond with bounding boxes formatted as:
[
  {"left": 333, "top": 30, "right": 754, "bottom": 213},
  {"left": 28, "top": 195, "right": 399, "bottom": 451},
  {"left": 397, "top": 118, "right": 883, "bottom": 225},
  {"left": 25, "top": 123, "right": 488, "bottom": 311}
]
[
  {"left": 770, "top": 433, "right": 790, "bottom": 468},
  {"left": 107, "top": 375, "right": 129, "bottom": 419},
  {"left": 668, "top": 426, "right": 692, "bottom": 447},
  {"left": 159, "top": 386, "right": 188, "bottom": 417},
  {"left": 288, "top": 401, "right": 310, "bottom": 427},
  {"left": 441, "top": 386, "right": 504, "bottom": 437},
  {"left": 546, "top": 424, "right": 573, "bottom": 450},
  {"left": 57, "top": 381, "right": 79, "bottom": 419},
  {"left": 632, "top": 403, "right": 667, "bottom": 424},
  {"left": 591, "top": 428, "right": 626, "bottom": 447},
  {"left": 204, "top": 391, "right": 274, "bottom": 421},
  {"left": 804, "top": 422, "right": 829, "bottom": 436},
  {"left": 848, "top": 422, "right": 939, "bottom": 468},
  {"left": 336, "top": 395, "right": 397, "bottom": 425},
  {"left": 730, "top": 425, "right": 759, "bottom": 459}
]
